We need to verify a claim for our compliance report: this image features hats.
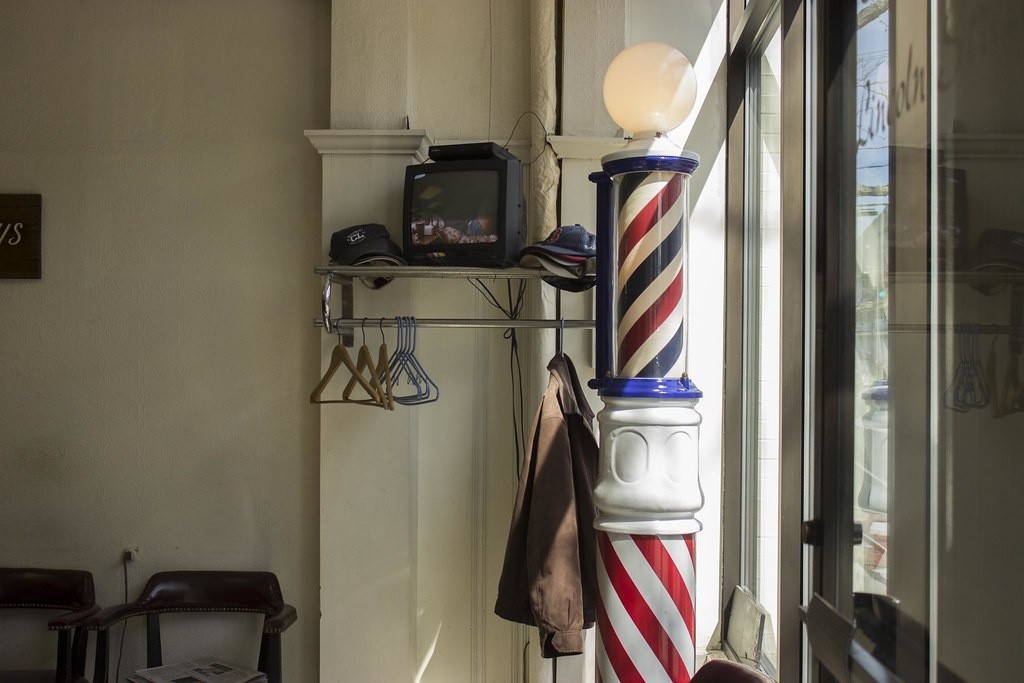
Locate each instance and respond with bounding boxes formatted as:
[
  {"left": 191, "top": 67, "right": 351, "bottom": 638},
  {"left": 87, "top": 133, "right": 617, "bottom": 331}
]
[
  {"left": 517, "top": 223, "right": 596, "bottom": 294},
  {"left": 328, "top": 223, "right": 407, "bottom": 290}
]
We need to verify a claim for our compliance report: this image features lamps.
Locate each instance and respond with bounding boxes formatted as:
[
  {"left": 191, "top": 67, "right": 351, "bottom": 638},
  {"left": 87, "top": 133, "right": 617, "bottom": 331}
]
[{"left": 593, "top": 40, "right": 699, "bottom": 683}]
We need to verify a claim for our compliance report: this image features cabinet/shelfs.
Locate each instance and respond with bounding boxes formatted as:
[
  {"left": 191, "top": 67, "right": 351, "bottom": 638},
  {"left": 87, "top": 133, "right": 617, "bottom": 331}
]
[{"left": 309, "top": 316, "right": 440, "bottom": 411}]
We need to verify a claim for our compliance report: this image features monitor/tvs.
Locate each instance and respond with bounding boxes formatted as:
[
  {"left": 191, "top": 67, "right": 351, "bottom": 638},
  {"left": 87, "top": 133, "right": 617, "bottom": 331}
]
[{"left": 403, "top": 162, "right": 528, "bottom": 266}]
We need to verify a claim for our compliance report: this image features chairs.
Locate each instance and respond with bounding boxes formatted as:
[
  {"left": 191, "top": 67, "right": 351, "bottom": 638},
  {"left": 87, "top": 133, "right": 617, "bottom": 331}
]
[
  {"left": 81, "top": 569, "right": 298, "bottom": 683},
  {"left": 0, "top": 567, "right": 96, "bottom": 683}
]
